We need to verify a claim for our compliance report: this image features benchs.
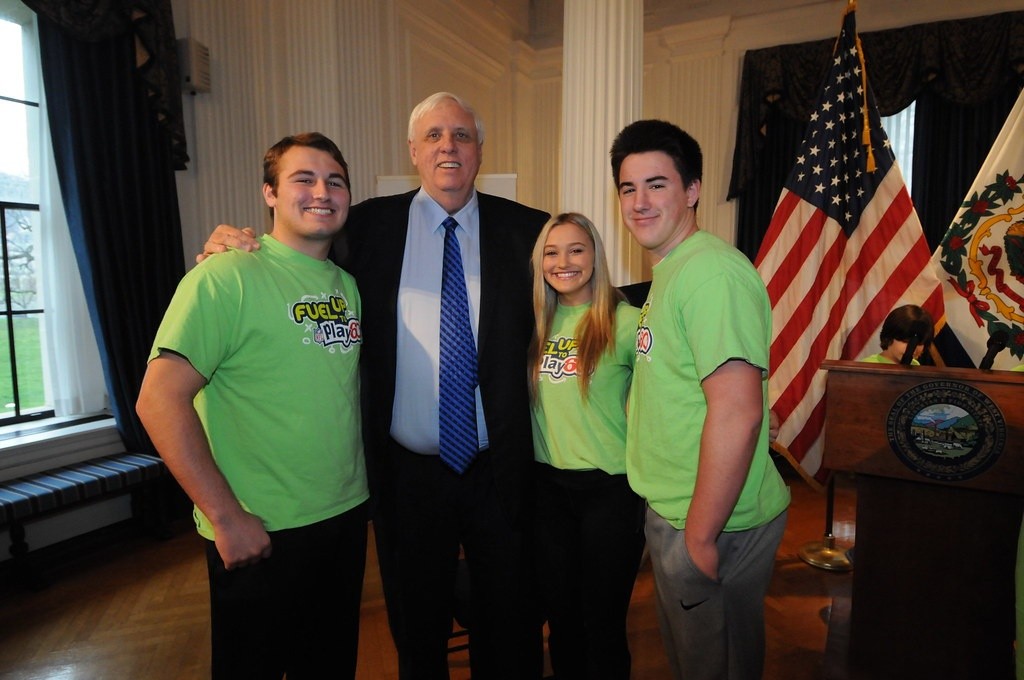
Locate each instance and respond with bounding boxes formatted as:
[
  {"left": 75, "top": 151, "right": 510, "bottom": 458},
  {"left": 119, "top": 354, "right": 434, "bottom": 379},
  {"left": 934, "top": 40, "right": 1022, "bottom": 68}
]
[{"left": 0, "top": 450, "right": 170, "bottom": 569}]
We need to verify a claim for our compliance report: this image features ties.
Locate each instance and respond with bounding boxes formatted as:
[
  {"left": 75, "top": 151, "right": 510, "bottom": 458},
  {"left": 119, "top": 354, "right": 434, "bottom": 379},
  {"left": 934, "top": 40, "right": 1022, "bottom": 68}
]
[{"left": 438, "top": 217, "right": 479, "bottom": 475}]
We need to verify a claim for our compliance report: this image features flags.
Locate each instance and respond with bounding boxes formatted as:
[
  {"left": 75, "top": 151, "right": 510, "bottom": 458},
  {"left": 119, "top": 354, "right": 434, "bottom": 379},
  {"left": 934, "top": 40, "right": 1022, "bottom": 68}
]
[
  {"left": 931, "top": 83, "right": 1024, "bottom": 377},
  {"left": 750, "top": 1, "right": 949, "bottom": 493}
]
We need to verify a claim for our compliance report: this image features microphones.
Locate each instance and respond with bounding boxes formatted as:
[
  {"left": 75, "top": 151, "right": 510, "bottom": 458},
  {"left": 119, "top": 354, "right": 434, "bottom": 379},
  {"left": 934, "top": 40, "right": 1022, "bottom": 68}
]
[
  {"left": 900, "top": 320, "right": 926, "bottom": 365},
  {"left": 979, "top": 330, "right": 1009, "bottom": 369}
]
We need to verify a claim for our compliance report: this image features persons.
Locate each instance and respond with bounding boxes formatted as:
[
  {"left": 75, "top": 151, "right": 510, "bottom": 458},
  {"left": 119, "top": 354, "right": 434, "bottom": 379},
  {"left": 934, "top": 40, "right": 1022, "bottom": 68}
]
[
  {"left": 194, "top": 90, "right": 553, "bottom": 680},
  {"left": 857, "top": 304, "right": 936, "bottom": 367},
  {"left": 525, "top": 213, "right": 647, "bottom": 680},
  {"left": 134, "top": 132, "right": 367, "bottom": 680},
  {"left": 610, "top": 120, "right": 791, "bottom": 680}
]
